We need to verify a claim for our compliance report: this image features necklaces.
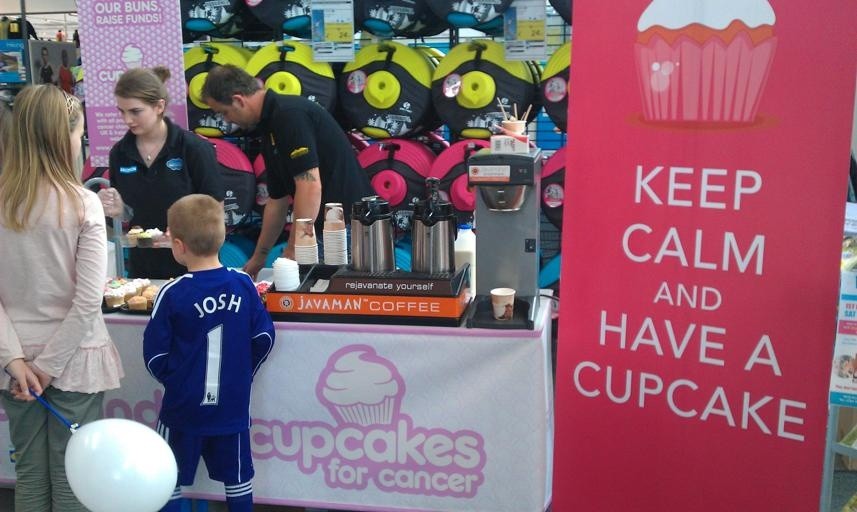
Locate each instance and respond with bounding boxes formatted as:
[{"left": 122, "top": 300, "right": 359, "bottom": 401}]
[{"left": 140, "top": 136, "right": 166, "bottom": 160}]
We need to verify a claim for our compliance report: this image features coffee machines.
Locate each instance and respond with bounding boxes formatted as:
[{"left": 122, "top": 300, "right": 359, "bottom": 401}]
[{"left": 467, "top": 146, "right": 544, "bottom": 332}]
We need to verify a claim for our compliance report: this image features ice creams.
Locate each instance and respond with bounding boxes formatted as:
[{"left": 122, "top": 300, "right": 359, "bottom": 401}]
[
  {"left": 315, "top": 344, "right": 407, "bottom": 427},
  {"left": 120, "top": 43, "right": 145, "bottom": 69}
]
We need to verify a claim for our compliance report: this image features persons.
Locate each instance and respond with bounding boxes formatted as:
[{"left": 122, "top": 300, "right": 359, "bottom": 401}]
[
  {"left": 0, "top": 83, "right": 125, "bottom": 511},
  {"left": 143, "top": 194, "right": 273, "bottom": 512},
  {"left": 197, "top": 64, "right": 372, "bottom": 283},
  {"left": 39, "top": 46, "right": 76, "bottom": 95},
  {"left": 55, "top": 29, "right": 64, "bottom": 41},
  {"left": 97, "top": 66, "right": 228, "bottom": 279},
  {"left": 73, "top": 29, "right": 80, "bottom": 48}
]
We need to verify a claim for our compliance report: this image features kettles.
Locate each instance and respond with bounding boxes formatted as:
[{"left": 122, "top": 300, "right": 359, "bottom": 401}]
[
  {"left": 409, "top": 176, "right": 459, "bottom": 277},
  {"left": 349, "top": 196, "right": 398, "bottom": 274}
]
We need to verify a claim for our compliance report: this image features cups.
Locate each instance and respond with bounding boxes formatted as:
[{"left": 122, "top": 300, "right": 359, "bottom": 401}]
[
  {"left": 293, "top": 201, "right": 347, "bottom": 267},
  {"left": 491, "top": 287, "right": 516, "bottom": 321}
]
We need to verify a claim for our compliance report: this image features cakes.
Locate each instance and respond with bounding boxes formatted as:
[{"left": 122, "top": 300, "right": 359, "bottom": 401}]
[
  {"left": 124, "top": 224, "right": 162, "bottom": 249},
  {"left": 633, "top": 0, "right": 778, "bottom": 125},
  {"left": 101, "top": 273, "right": 159, "bottom": 312}
]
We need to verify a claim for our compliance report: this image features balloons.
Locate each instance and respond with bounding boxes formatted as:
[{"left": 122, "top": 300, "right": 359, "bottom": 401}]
[{"left": 63, "top": 419, "right": 178, "bottom": 512}]
